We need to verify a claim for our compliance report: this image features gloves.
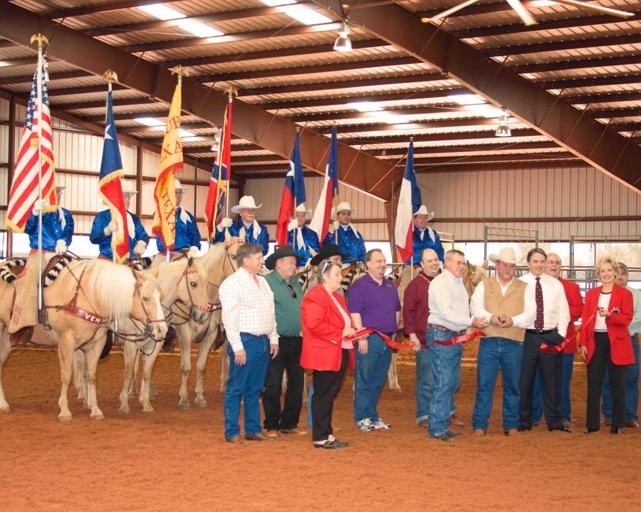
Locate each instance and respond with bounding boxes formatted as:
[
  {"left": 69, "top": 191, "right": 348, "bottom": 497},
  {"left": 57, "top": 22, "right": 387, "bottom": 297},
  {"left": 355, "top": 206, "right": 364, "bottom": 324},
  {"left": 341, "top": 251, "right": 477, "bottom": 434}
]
[
  {"left": 133, "top": 240, "right": 147, "bottom": 255},
  {"left": 287, "top": 217, "right": 298, "bottom": 232},
  {"left": 54, "top": 239, "right": 68, "bottom": 256},
  {"left": 217, "top": 218, "right": 232, "bottom": 232},
  {"left": 187, "top": 246, "right": 203, "bottom": 260},
  {"left": 328, "top": 221, "right": 338, "bottom": 234},
  {"left": 104, "top": 219, "right": 119, "bottom": 235},
  {"left": 32, "top": 199, "right": 48, "bottom": 215}
]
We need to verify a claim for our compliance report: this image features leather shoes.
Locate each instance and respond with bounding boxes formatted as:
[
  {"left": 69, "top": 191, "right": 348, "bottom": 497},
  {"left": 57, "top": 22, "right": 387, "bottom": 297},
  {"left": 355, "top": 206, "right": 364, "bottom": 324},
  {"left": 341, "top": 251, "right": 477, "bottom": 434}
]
[
  {"left": 610, "top": 425, "right": 619, "bottom": 436},
  {"left": 267, "top": 429, "right": 280, "bottom": 438},
  {"left": 333, "top": 440, "right": 348, "bottom": 448},
  {"left": 472, "top": 428, "right": 486, "bottom": 437},
  {"left": 228, "top": 435, "right": 249, "bottom": 445},
  {"left": 604, "top": 416, "right": 612, "bottom": 425},
  {"left": 316, "top": 440, "right": 336, "bottom": 450},
  {"left": 435, "top": 432, "right": 462, "bottom": 441},
  {"left": 553, "top": 425, "right": 572, "bottom": 432},
  {"left": 629, "top": 420, "right": 640, "bottom": 428},
  {"left": 418, "top": 419, "right": 430, "bottom": 428},
  {"left": 281, "top": 428, "right": 309, "bottom": 436},
  {"left": 519, "top": 423, "right": 533, "bottom": 432},
  {"left": 584, "top": 425, "right": 600, "bottom": 434},
  {"left": 506, "top": 428, "right": 520, "bottom": 437},
  {"left": 245, "top": 432, "right": 267, "bottom": 441},
  {"left": 449, "top": 412, "right": 466, "bottom": 424}
]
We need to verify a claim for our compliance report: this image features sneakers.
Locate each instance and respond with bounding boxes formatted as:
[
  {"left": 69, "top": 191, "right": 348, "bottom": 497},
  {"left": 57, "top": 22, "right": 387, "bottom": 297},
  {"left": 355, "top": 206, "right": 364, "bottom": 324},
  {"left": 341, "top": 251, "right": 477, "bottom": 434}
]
[
  {"left": 356, "top": 418, "right": 376, "bottom": 433},
  {"left": 375, "top": 419, "right": 392, "bottom": 430}
]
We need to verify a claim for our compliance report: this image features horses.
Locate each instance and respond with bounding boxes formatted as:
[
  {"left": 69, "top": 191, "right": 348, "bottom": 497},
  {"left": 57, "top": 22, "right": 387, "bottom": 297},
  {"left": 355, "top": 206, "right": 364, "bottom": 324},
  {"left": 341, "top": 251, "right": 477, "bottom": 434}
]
[
  {"left": 1, "top": 254, "right": 169, "bottom": 420},
  {"left": 126, "top": 227, "right": 247, "bottom": 408},
  {"left": 385, "top": 260, "right": 492, "bottom": 393},
  {"left": 69, "top": 252, "right": 210, "bottom": 414},
  {"left": 279, "top": 245, "right": 319, "bottom": 400}
]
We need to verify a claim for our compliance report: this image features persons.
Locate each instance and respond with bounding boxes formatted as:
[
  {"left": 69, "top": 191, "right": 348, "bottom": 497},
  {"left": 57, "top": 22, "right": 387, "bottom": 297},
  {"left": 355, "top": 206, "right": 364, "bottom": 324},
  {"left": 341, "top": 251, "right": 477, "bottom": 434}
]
[
  {"left": 600, "top": 263, "right": 640, "bottom": 427},
  {"left": 545, "top": 253, "right": 585, "bottom": 432},
  {"left": 404, "top": 204, "right": 444, "bottom": 269},
  {"left": 326, "top": 199, "right": 366, "bottom": 262},
  {"left": 404, "top": 248, "right": 465, "bottom": 431},
  {"left": 156, "top": 178, "right": 201, "bottom": 256},
  {"left": 578, "top": 259, "right": 633, "bottom": 435},
  {"left": 516, "top": 249, "right": 570, "bottom": 434},
  {"left": 217, "top": 243, "right": 278, "bottom": 443},
  {"left": 285, "top": 202, "right": 320, "bottom": 267},
  {"left": 426, "top": 251, "right": 493, "bottom": 440},
  {"left": 26, "top": 184, "right": 73, "bottom": 257},
  {"left": 89, "top": 182, "right": 148, "bottom": 266},
  {"left": 300, "top": 260, "right": 368, "bottom": 450},
  {"left": 470, "top": 248, "right": 535, "bottom": 438},
  {"left": 349, "top": 249, "right": 402, "bottom": 434},
  {"left": 263, "top": 244, "right": 306, "bottom": 438},
  {"left": 211, "top": 195, "right": 269, "bottom": 257}
]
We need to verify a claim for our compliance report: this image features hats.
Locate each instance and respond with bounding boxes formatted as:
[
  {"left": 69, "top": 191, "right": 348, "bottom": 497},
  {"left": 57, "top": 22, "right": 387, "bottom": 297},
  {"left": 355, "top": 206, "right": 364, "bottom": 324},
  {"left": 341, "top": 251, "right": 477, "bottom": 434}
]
[
  {"left": 488, "top": 248, "right": 519, "bottom": 265},
  {"left": 121, "top": 181, "right": 139, "bottom": 194},
  {"left": 329, "top": 201, "right": 356, "bottom": 219},
  {"left": 231, "top": 195, "right": 262, "bottom": 213},
  {"left": 175, "top": 179, "right": 189, "bottom": 194},
  {"left": 311, "top": 243, "right": 346, "bottom": 263},
  {"left": 414, "top": 205, "right": 435, "bottom": 221},
  {"left": 295, "top": 203, "right": 313, "bottom": 221},
  {"left": 265, "top": 244, "right": 303, "bottom": 269},
  {"left": 54, "top": 181, "right": 68, "bottom": 190}
]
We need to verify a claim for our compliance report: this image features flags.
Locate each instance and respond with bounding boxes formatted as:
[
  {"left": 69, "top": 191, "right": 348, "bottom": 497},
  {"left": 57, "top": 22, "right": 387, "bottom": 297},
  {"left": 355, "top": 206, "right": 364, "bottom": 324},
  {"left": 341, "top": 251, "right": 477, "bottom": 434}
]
[
  {"left": 278, "top": 128, "right": 304, "bottom": 250},
  {"left": 203, "top": 96, "right": 233, "bottom": 232},
  {"left": 98, "top": 78, "right": 130, "bottom": 266},
  {"left": 150, "top": 70, "right": 182, "bottom": 253},
  {"left": 7, "top": 47, "right": 55, "bottom": 235},
  {"left": 310, "top": 126, "right": 339, "bottom": 244},
  {"left": 395, "top": 141, "right": 420, "bottom": 261}
]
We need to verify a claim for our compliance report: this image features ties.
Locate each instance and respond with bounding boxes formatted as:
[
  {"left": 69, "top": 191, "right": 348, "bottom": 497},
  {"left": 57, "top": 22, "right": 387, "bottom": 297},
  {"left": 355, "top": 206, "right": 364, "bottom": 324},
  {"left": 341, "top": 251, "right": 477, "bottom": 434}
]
[{"left": 535, "top": 277, "right": 544, "bottom": 333}]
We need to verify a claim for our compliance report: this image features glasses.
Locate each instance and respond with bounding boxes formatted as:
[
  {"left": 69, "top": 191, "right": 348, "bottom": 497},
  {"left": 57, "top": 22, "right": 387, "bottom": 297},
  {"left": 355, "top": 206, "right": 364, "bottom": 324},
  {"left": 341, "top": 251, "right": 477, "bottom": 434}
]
[
  {"left": 287, "top": 284, "right": 297, "bottom": 298},
  {"left": 322, "top": 260, "right": 343, "bottom": 274}
]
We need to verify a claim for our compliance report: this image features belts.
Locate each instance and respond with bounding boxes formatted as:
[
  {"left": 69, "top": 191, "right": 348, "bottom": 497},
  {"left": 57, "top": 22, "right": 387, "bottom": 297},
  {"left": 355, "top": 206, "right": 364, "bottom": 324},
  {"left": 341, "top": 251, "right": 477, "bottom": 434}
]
[
  {"left": 433, "top": 325, "right": 465, "bottom": 334},
  {"left": 526, "top": 328, "right": 559, "bottom": 334}
]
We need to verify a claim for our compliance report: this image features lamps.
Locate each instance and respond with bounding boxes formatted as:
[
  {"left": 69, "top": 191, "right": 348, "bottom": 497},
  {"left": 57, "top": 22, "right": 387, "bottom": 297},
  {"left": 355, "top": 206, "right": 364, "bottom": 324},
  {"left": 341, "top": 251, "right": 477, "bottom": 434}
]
[
  {"left": 495, "top": 106, "right": 514, "bottom": 138},
  {"left": 333, "top": 2, "right": 354, "bottom": 54}
]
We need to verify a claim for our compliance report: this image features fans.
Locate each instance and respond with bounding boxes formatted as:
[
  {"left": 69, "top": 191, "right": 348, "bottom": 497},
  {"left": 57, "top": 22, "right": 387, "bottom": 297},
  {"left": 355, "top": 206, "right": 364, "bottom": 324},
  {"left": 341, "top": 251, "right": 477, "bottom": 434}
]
[{"left": 431, "top": 0, "right": 636, "bottom": 26}]
